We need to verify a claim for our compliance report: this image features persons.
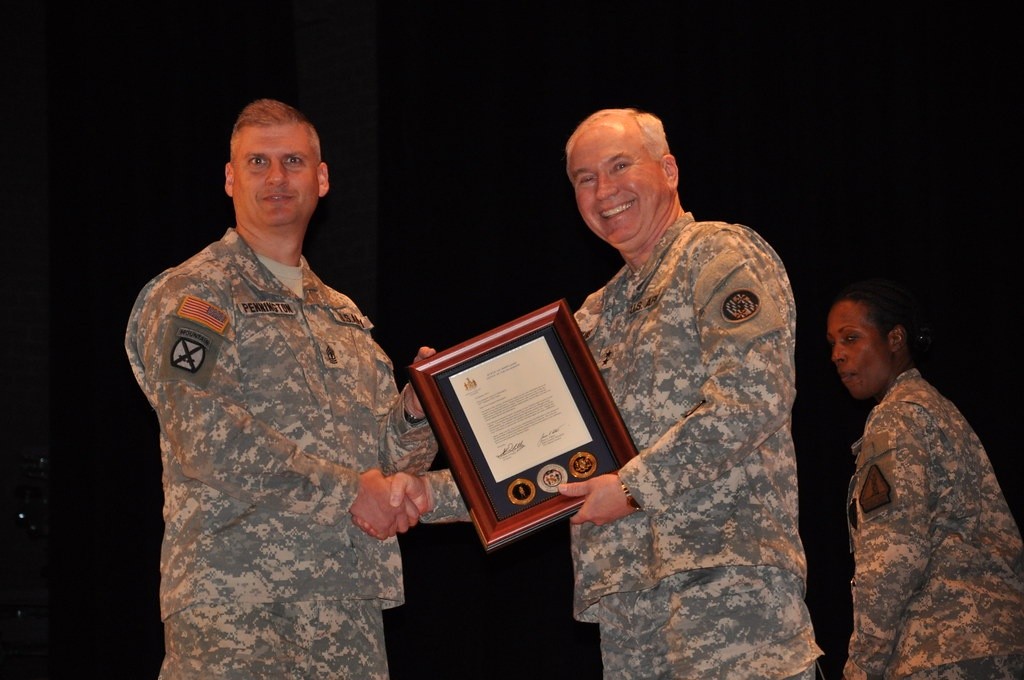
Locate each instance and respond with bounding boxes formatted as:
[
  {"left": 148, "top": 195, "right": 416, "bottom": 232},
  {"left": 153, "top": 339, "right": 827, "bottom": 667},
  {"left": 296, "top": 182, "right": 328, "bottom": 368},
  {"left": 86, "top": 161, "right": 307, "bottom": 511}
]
[
  {"left": 125, "top": 99, "right": 436, "bottom": 680},
  {"left": 827, "top": 279, "right": 1024, "bottom": 680},
  {"left": 351, "top": 108, "right": 826, "bottom": 680}
]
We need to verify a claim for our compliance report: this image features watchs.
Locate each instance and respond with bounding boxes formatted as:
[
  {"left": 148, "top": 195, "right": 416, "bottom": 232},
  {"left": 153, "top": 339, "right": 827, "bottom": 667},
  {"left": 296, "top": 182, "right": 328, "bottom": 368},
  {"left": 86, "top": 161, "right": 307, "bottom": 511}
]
[{"left": 619, "top": 481, "right": 643, "bottom": 513}]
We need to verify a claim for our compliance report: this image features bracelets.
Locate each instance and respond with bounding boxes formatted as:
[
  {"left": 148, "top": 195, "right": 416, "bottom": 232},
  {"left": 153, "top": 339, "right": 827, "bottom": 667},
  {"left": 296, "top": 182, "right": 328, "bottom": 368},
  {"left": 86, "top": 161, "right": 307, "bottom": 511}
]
[{"left": 403, "top": 408, "right": 423, "bottom": 424}]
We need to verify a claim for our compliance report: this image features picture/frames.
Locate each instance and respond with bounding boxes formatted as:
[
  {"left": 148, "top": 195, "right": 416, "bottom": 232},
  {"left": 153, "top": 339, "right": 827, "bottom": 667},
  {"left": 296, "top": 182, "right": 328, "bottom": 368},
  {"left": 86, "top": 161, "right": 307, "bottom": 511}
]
[{"left": 409, "top": 291, "right": 640, "bottom": 554}]
